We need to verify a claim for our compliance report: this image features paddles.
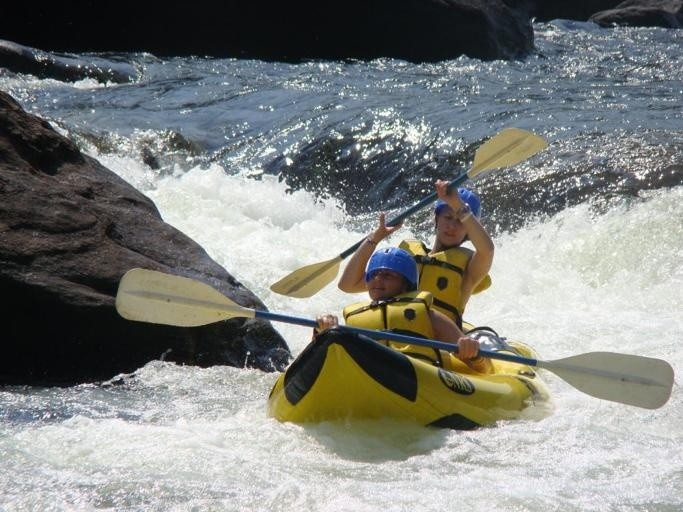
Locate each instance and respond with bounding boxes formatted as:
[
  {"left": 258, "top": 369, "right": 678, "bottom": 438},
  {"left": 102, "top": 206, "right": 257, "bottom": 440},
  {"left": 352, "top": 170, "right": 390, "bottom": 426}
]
[
  {"left": 271, "top": 126, "right": 548, "bottom": 298},
  {"left": 116, "top": 268, "right": 675, "bottom": 410}
]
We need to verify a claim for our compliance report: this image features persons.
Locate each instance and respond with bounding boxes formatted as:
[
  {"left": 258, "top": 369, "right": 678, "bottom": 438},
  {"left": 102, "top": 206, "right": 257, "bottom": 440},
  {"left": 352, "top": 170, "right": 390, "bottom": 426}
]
[{"left": 313, "top": 179, "right": 495, "bottom": 375}]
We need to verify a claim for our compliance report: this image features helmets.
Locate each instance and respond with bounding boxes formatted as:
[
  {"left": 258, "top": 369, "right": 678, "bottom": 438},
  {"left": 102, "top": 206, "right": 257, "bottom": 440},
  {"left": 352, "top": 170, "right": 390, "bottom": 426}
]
[
  {"left": 435, "top": 187, "right": 481, "bottom": 217},
  {"left": 365, "top": 246, "right": 419, "bottom": 291}
]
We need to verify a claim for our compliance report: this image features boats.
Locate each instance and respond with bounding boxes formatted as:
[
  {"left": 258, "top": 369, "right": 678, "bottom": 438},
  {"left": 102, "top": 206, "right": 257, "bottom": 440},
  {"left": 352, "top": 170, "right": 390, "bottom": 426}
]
[{"left": 265, "top": 320, "right": 555, "bottom": 430}]
